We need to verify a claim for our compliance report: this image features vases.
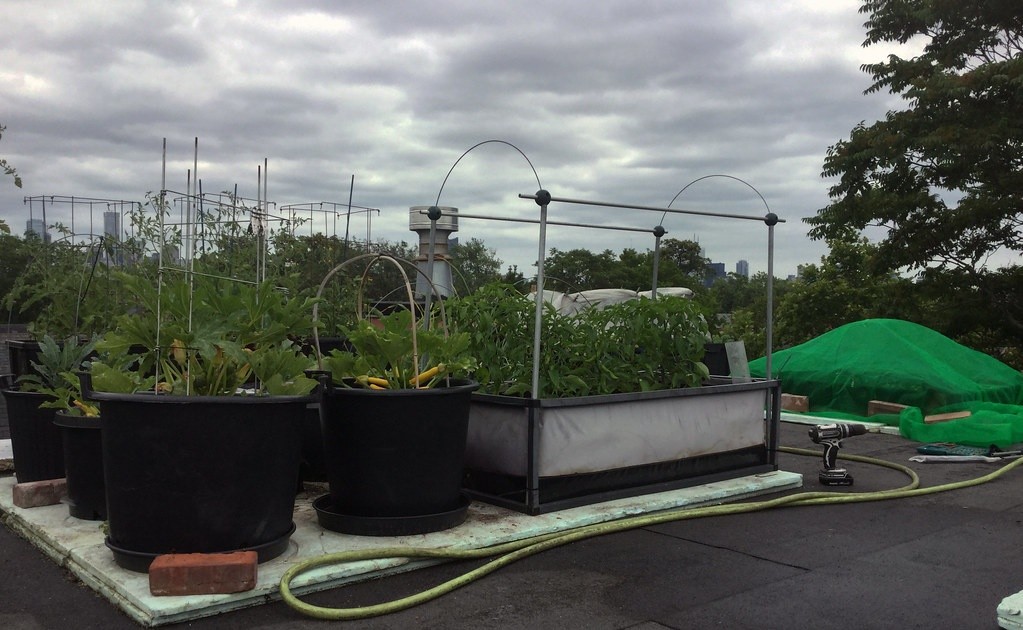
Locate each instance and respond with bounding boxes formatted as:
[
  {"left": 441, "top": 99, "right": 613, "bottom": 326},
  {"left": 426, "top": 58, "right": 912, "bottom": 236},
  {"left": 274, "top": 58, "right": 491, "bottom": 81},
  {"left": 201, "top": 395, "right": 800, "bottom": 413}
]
[
  {"left": 320, "top": 377, "right": 477, "bottom": 514},
  {"left": 100, "top": 400, "right": 310, "bottom": 552},
  {"left": 50, "top": 422, "right": 108, "bottom": 521}
]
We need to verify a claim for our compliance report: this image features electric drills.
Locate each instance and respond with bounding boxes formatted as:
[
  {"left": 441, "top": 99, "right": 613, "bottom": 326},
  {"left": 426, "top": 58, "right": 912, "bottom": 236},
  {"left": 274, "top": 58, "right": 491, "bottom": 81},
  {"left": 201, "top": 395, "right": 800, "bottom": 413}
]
[{"left": 808, "top": 422, "right": 889, "bottom": 486}]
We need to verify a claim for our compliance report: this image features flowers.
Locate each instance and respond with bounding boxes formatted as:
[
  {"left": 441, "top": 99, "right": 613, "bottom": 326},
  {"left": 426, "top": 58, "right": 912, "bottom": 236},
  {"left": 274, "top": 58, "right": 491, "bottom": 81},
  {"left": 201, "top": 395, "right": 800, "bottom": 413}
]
[
  {"left": 89, "top": 330, "right": 319, "bottom": 397},
  {"left": 35, "top": 372, "right": 100, "bottom": 417},
  {"left": 322, "top": 306, "right": 476, "bottom": 386}
]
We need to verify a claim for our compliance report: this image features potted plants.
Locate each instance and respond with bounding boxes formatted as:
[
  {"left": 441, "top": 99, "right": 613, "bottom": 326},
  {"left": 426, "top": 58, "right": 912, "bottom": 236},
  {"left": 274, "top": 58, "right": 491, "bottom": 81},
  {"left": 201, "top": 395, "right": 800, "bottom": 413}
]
[
  {"left": 0, "top": 337, "right": 79, "bottom": 483},
  {"left": 474, "top": 284, "right": 779, "bottom": 515},
  {"left": 8, "top": 321, "right": 65, "bottom": 386}
]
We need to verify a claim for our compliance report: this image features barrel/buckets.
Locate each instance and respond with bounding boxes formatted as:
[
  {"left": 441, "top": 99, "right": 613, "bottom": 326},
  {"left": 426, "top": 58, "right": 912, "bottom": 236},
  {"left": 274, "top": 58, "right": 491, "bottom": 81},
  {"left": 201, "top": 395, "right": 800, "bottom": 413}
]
[{"left": 702, "top": 344, "right": 731, "bottom": 376}]
